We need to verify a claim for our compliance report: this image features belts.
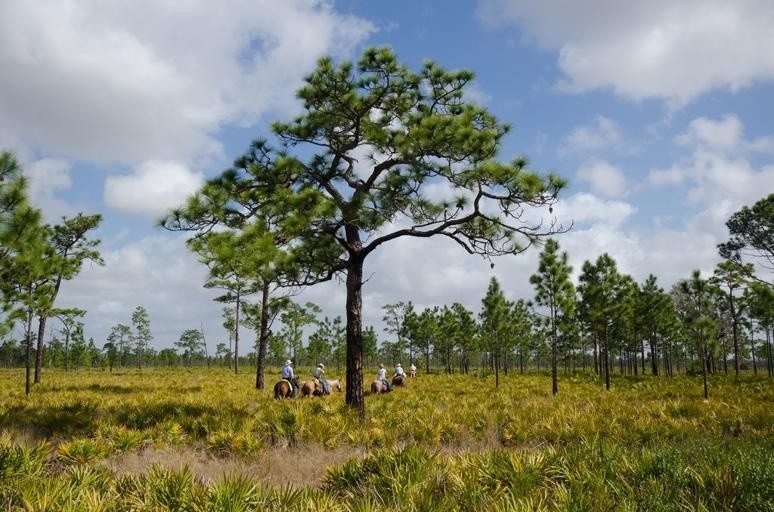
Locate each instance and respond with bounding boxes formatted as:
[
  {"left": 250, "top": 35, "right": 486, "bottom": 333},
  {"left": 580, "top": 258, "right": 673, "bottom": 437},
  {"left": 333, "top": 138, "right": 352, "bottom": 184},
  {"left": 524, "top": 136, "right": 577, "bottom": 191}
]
[
  {"left": 280, "top": 378, "right": 290, "bottom": 379},
  {"left": 314, "top": 376, "right": 320, "bottom": 378}
]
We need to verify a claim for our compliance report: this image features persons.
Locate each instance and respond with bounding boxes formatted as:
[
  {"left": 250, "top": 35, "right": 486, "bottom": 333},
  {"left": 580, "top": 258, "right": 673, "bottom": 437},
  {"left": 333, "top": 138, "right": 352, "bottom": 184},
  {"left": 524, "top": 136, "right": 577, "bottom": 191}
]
[
  {"left": 377, "top": 363, "right": 394, "bottom": 392},
  {"left": 409, "top": 363, "right": 417, "bottom": 371},
  {"left": 282, "top": 359, "right": 297, "bottom": 399},
  {"left": 314, "top": 363, "right": 331, "bottom": 395},
  {"left": 395, "top": 363, "right": 405, "bottom": 378}
]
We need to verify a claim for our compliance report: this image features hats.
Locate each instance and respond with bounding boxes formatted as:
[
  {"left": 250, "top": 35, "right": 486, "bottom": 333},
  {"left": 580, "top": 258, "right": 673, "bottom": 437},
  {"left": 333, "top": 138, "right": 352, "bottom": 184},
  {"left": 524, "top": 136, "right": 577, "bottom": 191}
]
[
  {"left": 317, "top": 363, "right": 324, "bottom": 369},
  {"left": 379, "top": 364, "right": 384, "bottom": 369},
  {"left": 284, "top": 359, "right": 293, "bottom": 366},
  {"left": 395, "top": 363, "right": 401, "bottom": 367}
]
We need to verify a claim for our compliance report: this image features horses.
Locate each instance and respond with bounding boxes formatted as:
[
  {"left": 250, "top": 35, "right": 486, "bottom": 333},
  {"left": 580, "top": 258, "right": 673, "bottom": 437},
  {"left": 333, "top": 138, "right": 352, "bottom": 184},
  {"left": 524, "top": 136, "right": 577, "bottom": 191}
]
[
  {"left": 391, "top": 375, "right": 406, "bottom": 387},
  {"left": 370, "top": 379, "right": 389, "bottom": 396},
  {"left": 408, "top": 371, "right": 416, "bottom": 378},
  {"left": 300, "top": 376, "right": 341, "bottom": 399},
  {"left": 274, "top": 376, "right": 299, "bottom": 402}
]
